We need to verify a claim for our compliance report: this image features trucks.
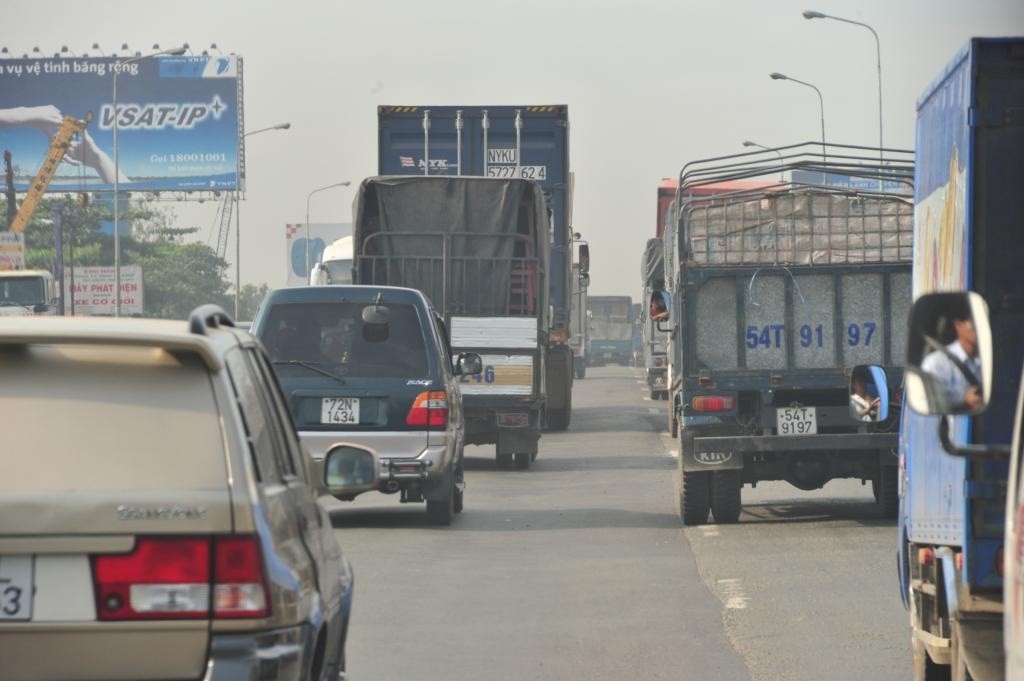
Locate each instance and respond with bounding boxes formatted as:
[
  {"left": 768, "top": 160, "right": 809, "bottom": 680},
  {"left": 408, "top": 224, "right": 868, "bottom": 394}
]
[
  {"left": 308, "top": 235, "right": 355, "bottom": 285},
  {"left": 376, "top": 104, "right": 591, "bottom": 434},
  {"left": 349, "top": 172, "right": 570, "bottom": 471},
  {"left": 1, "top": 269, "right": 63, "bottom": 317},
  {"left": 846, "top": 36, "right": 1024, "bottom": 681},
  {"left": 586, "top": 237, "right": 670, "bottom": 401},
  {"left": 647, "top": 141, "right": 914, "bottom": 526}
]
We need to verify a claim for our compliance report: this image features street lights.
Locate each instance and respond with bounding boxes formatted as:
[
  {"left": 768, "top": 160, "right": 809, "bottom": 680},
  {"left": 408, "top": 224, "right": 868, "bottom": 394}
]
[
  {"left": 112, "top": 44, "right": 190, "bottom": 316},
  {"left": 771, "top": 72, "right": 829, "bottom": 187},
  {"left": 803, "top": 10, "right": 885, "bottom": 192},
  {"left": 305, "top": 180, "right": 352, "bottom": 287},
  {"left": 743, "top": 139, "right": 785, "bottom": 182},
  {"left": 234, "top": 123, "right": 292, "bottom": 322}
]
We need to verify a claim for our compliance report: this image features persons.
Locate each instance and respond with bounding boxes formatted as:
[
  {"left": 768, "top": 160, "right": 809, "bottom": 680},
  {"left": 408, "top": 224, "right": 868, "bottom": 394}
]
[
  {"left": 852, "top": 375, "right": 883, "bottom": 421},
  {"left": 923, "top": 296, "right": 987, "bottom": 415},
  {"left": 651, "top": 300, "right": 669, "bottom": 320},
  {"left": 0, "top": 105, "right": 132, "bottom": 186}
]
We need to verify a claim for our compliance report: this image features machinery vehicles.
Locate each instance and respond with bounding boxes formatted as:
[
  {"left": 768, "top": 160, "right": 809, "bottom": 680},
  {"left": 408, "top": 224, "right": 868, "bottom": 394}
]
[{"left": 0, "top": 110, "right": 95, "bottom": 268}]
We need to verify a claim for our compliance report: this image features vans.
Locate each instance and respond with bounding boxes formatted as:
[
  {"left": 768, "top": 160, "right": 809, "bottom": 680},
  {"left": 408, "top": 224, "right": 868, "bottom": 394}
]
[{"left": 246, "top": 285, "right": 484, "bottom": 527}]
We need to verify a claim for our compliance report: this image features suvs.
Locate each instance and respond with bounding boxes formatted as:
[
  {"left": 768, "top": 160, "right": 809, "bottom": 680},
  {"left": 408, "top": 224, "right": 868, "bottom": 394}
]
[{"left": 0, "top": 300, "right": 383, "bottom": 681}]
